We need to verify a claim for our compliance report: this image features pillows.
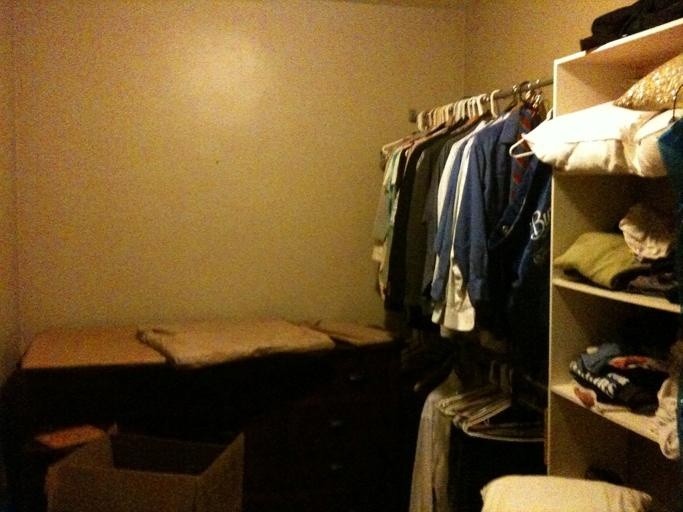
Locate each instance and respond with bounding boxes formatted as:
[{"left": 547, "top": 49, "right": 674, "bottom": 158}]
[{"left": 520, "top": 53, "right": 683, "bottom": 178}]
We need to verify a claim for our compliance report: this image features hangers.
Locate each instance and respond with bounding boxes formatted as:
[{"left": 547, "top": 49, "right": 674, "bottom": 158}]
[
  {"left": 379, "top": 79, "right": 556, "bottom": 161},
  {"left": 435, "top": 362, "right": 547, "bottom": 444}
]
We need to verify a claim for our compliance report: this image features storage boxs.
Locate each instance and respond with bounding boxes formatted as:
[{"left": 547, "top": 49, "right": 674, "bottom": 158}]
[{"left": 38, "top": 428, "right": 244, "bottom": 512}]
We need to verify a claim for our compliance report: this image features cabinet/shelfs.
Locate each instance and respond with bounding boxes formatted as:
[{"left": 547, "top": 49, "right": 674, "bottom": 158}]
[{"left": 546, "top": 19, "right": 683, "bottom": 511}]
